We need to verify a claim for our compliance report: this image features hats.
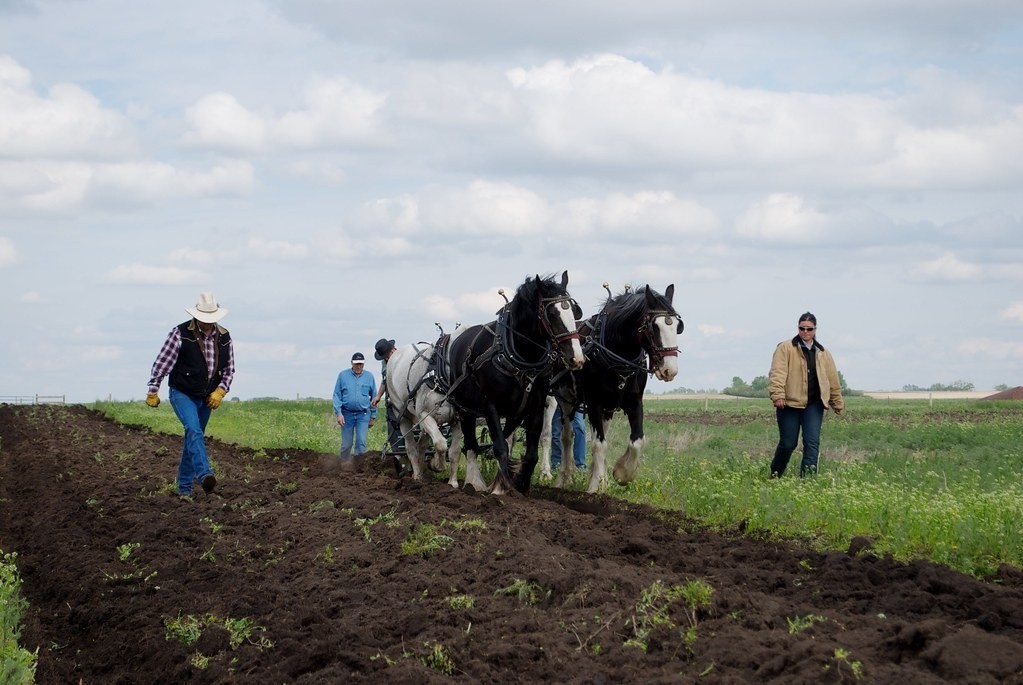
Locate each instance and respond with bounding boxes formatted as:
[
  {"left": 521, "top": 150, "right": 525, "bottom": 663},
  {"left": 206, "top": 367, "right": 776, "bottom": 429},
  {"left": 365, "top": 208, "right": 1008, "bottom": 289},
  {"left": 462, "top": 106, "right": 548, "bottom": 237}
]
[
  {"left": 351, "top": 352, "right": 365, "bottom": 364},
  {"left": 374, "top": 338, "right": 395, "bottom": 360},
  {"left": 185, "top": 292, "right": 228, "bottom": 323}
]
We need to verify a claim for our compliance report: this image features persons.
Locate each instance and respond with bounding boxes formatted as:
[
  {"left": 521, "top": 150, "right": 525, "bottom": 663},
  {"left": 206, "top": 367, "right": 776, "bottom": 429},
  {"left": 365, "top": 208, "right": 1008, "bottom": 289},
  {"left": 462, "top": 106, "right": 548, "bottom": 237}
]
[
  {"left": 370, "top": 338, "right": 406, "bottom": 454},
  {"left": 332, "top": 351, "right": 377, "bottom": 460},
  {"left": 146, "top": 292, "right": 235, "bottom": 503},
  {"left": 551, "top": 404, "right": 588, "bottom": 475},
  {"left": 768, "top": 312, "right": 845, "bottom": 477}
]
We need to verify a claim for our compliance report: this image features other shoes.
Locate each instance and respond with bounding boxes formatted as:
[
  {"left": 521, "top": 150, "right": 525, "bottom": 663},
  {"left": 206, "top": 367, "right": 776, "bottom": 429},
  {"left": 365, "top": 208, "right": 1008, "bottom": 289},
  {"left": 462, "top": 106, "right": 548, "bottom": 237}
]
[
  {"left": 551, "top": 464, "right": 560, "bottom": 470},
  {"left": 576, "top": 464, "right": 587, "bottom": 471},
  {"left": 201, "top": 475, "right": 217, "bottom": 497}
]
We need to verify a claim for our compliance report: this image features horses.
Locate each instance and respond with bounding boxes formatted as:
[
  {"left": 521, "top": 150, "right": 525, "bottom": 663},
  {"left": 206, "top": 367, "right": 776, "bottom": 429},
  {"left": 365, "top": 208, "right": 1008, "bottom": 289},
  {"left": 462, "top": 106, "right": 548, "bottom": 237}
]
[{"left": 386, "top": 270, "right": 686, "bottom": 498}]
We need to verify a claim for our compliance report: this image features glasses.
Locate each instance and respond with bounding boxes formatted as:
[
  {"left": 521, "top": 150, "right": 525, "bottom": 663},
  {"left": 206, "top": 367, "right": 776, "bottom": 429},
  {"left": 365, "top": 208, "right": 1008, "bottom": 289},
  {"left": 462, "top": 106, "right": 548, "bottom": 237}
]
[{"left": 798, "top": 327, "right": 815, "bottom": 331}]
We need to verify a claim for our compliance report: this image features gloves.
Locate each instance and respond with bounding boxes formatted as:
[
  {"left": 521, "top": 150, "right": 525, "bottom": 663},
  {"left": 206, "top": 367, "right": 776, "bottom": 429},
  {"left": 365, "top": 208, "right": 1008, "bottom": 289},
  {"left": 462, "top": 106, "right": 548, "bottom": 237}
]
[
  {"left": 205, "top": 386, "right": 226, "bottom": 410},
  {"left": 146, "top": 393, "right": 160, "bottom": 407}
]
